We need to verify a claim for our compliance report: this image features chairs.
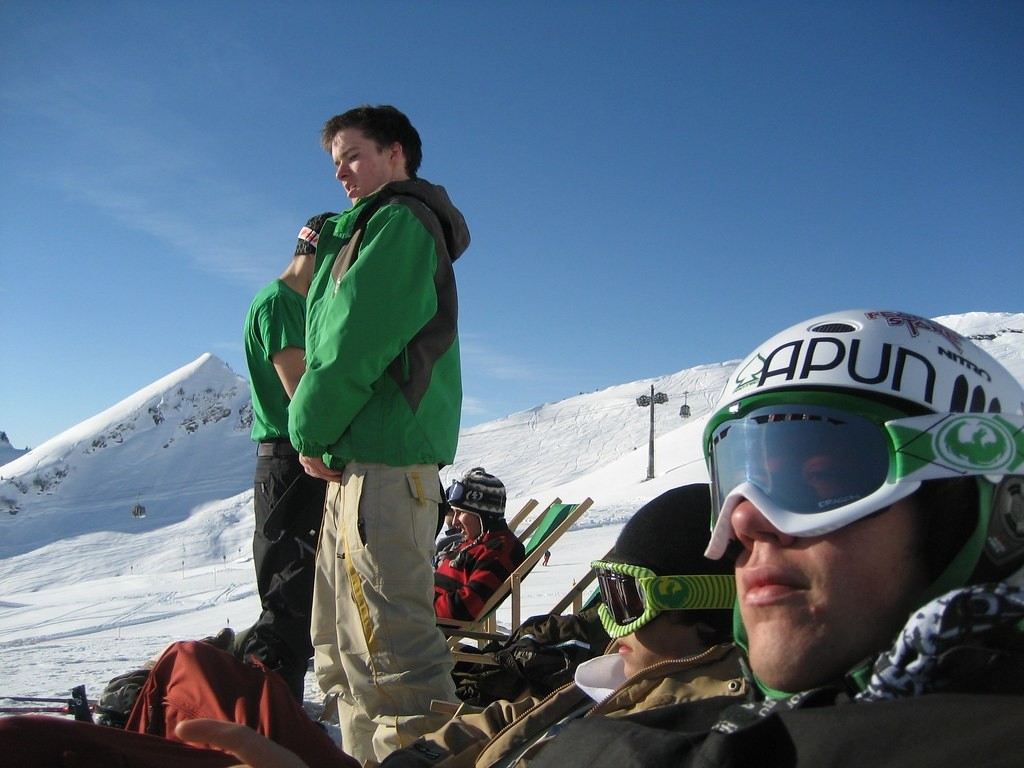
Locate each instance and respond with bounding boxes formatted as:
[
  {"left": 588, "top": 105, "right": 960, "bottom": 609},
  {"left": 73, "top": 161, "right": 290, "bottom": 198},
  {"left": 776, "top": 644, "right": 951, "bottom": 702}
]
[{"left": 430, "top": 497, "right": 616, "bottom": 723}]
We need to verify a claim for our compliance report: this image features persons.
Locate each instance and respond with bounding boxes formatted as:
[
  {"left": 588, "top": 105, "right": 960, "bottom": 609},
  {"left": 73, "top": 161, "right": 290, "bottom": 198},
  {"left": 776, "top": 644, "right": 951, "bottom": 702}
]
[
  {"left": 1, "top": 310, "right": 1024, "bottom": 768},
  {"left": 287, "top": 104, "right": 473, "bottom": 768},
  {"left": 230, "top": 213, "right": 344, "bottom": 706}
]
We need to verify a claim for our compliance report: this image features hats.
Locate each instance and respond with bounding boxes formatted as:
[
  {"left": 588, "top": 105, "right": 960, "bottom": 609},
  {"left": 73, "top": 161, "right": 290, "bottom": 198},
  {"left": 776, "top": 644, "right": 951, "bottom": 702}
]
[
  {"left": 449, "top": 467, "right": 506, "bottom": 567},
  {"left": 294, "top": 212, "right": 340, "bottom": 254},
  {"left": 617, "top": 482, "right": 735, "bottom": 625}
]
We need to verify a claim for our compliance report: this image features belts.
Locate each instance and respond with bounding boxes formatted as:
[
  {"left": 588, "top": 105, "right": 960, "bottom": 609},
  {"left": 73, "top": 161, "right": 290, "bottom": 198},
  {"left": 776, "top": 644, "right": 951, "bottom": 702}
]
[{"left": 256, "top": 442, "right": 296, "bottom": 456}]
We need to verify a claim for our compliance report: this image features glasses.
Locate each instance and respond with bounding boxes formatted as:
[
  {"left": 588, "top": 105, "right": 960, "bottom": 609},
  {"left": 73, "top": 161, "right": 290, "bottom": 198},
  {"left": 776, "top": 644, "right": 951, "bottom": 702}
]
[
  {"left": 702, "top": 391, "right": 913, "bottom": 560},
  {"left": 590, "top": 560, "right": 662, "bottom": 637},
  {"left": 447, "top": 479, "right": 469, "bottom": 504}
]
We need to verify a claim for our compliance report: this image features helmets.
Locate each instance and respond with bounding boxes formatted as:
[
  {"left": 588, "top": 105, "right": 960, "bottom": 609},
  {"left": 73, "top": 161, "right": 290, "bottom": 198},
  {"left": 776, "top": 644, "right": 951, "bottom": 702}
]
[{"left": 707, "top": 307, "right": 1024, "bottom": 566}]
